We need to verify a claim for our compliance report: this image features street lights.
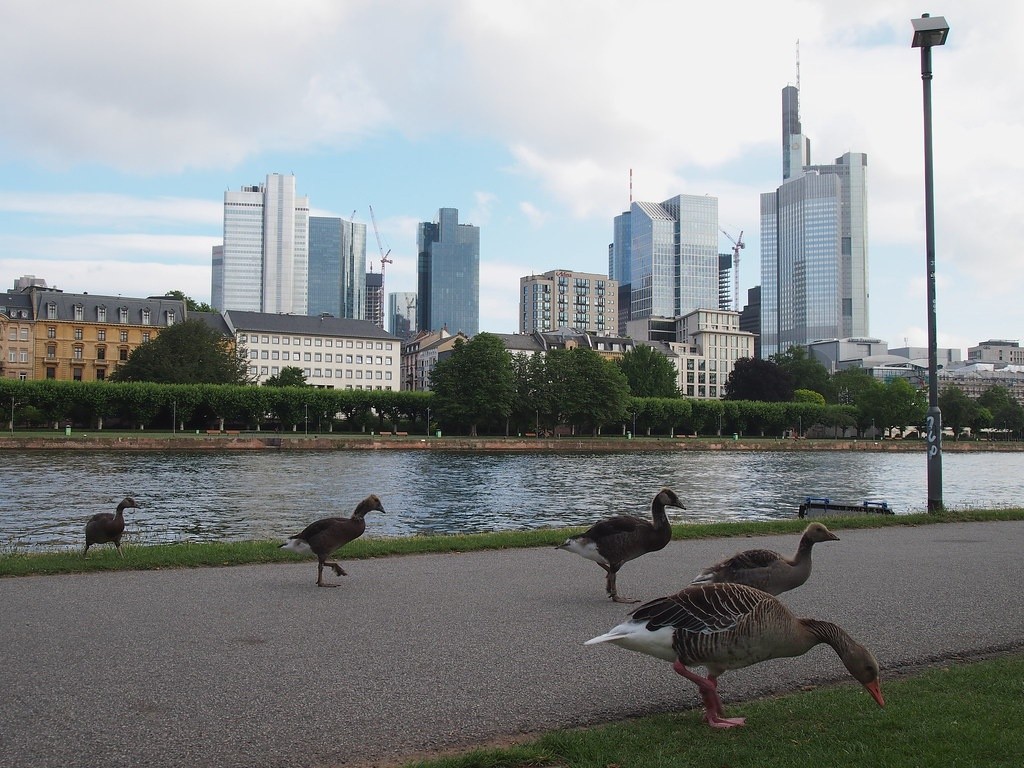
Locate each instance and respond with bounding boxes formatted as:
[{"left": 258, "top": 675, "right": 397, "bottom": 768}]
[
  {"left": 718, "top": 413, "right": 721, "bottom": 437},
  {"left": 872, "top": 418, "right": 875, "bottom": 441},
  {"left": 633, "top": 411, "right": 636, "bottom": 437},
  {"left": 172, "top": 401, "right": 176, "bottom": 436},
  {"left": 799, "top": 415, "right": 801, "bottom": 439},
  {"left": 11, "top": 397, "right": 21, "bottom": 437},
  {"left": 304, "top": 404, "right": 309, "bottom": 435},
  {"left": 909, "top": 12, "right": 951, "bottom": 515},
  {"left": 426, "top": 407, "right": 431, "bottom": 438},
  {"left": 536, "top": 409, "right": 539, "bottom": 439},
  {"left": 1003, "top": 421, "right": 1007, "bottom": 441}
]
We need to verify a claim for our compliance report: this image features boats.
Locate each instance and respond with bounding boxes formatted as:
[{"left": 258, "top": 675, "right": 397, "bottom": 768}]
[{"left": 798, "top": 495, "right": 895, "bottom": 519}]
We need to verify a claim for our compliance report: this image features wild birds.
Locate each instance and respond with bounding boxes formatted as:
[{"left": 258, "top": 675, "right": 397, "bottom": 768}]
[
  {"left": 82, "top": 497, "right": 142, "bottom": 560},
  {"left": 583, "top": 581, "right": 885, "bottom": 730},
  {"left": 554, "top": 488, "right": 686, "bottom": 604},
  {"left": 689, "top": 521, "right": 841, "bottom": 598},
  {"left": 276, "top": 494, "right": 386, "bottom": 588}
]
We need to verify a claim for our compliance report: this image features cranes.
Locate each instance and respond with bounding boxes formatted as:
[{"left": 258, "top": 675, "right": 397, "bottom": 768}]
[
  {"left": 719, "top": 226, "right": 745, "bottom": 313},
  {"left": 406, "top": 298, "right": 417, "bottom": 340},
  {"left": 368, "top": 205, "right": 393, "bottom": 330}
]
